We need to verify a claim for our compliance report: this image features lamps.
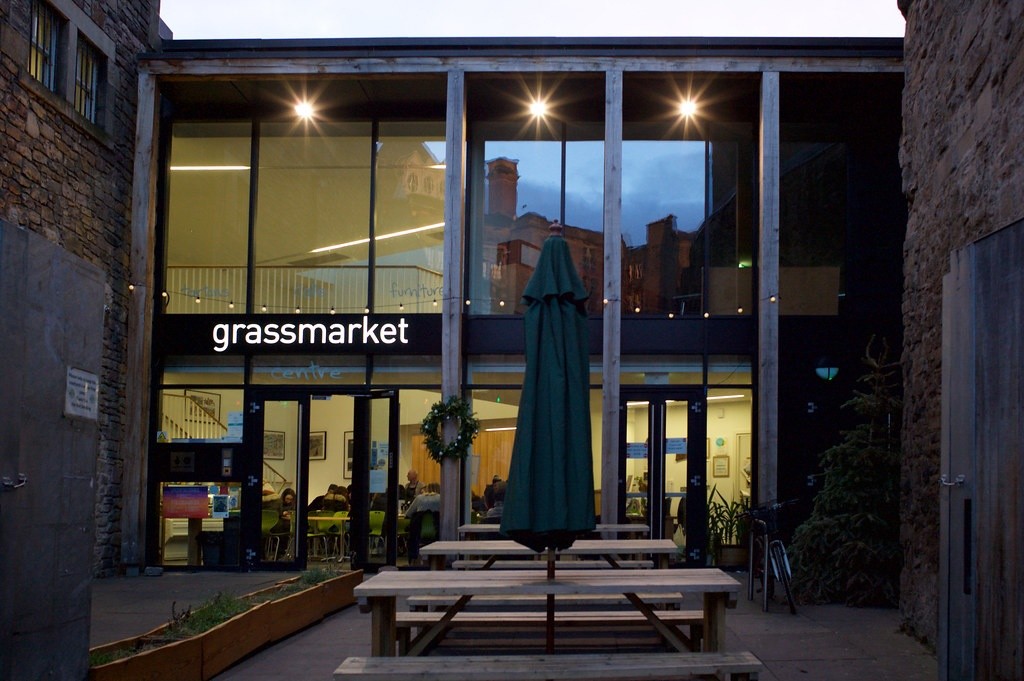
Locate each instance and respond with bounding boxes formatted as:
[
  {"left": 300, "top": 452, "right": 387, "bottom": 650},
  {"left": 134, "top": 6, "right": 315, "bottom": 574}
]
[{"left": 814, "top": 351, "right": 840, "bottom": 380}]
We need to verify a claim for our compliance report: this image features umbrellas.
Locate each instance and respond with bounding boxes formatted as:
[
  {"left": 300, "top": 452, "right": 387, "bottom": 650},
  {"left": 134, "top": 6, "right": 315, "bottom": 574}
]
[{"left": 498, "top": 223, "right": 597, "bottom": 655}]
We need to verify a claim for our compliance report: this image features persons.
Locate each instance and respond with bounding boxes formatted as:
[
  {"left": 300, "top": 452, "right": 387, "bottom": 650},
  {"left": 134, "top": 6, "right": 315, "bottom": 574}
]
[
  {"left": 270, "top": 488, "right": 296, "bottom": 560},
  {"left": 472, "top": 475, "right": 509, "bottom": 516},
  {"left": 308, "top": 483, "right": 372, "bottom": 558},
  {"left": 399, "top": 470, "right": 441, "bottom": 531}
]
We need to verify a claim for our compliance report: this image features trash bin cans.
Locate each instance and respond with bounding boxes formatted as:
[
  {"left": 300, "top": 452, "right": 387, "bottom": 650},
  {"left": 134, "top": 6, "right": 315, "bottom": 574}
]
[
  {"left": 199, "top": 531, "right": 225, "bottom": 568},
  {"left": 223, "top": 512, "right": 242, "bottom": 566}
]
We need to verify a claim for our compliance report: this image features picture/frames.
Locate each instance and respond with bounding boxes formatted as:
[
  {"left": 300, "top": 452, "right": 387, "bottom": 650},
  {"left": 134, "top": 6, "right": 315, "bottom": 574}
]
[
  {"left": 263, "top": 430, "right": 285, "bottom": 460},
  {"left": 309, "top": 430, "right": 326, "bottom": 460},
  {"left": 713, "top": 455, "right": 729, "bottom": 477},
  {"left": 707, "top": 438, "right": 710, "bottom": 458},
  {"left": 343, "top": 430, "right": 353, "bottom": 479}
]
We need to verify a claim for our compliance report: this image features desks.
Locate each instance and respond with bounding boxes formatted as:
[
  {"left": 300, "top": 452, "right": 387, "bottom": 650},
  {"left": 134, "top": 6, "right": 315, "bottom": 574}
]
[
  {"left": 354, "top": 569, "right": 741, "bottom": 681},
  {"left": 457, "top": 524, "right": 649, "bottom": 538},
  {"left": 420, "top": 540, "right": 678, "bottom": 569}
]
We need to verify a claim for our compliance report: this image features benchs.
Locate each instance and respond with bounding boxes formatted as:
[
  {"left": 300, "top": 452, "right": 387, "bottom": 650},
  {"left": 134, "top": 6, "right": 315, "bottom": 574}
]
[{"left": 336, "top": 559, "right": 764, "bottom": 681}]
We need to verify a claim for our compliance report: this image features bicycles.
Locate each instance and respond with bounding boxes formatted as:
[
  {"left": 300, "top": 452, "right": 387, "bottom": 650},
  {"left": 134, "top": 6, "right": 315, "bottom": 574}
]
[{"left": 733, "top": 496, "right": 801, "bottom": 616}]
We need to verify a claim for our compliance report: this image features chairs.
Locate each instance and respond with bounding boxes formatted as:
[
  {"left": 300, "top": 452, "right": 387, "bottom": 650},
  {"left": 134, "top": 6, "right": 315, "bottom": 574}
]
[{"left": 235, "top": 505, "right": 413, "bottom": 565}]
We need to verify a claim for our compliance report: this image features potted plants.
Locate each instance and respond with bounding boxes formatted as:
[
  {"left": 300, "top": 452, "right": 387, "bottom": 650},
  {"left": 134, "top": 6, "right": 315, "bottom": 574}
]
[{"left": 86, "top": 564, "right": 363, "bottom": 681}]
